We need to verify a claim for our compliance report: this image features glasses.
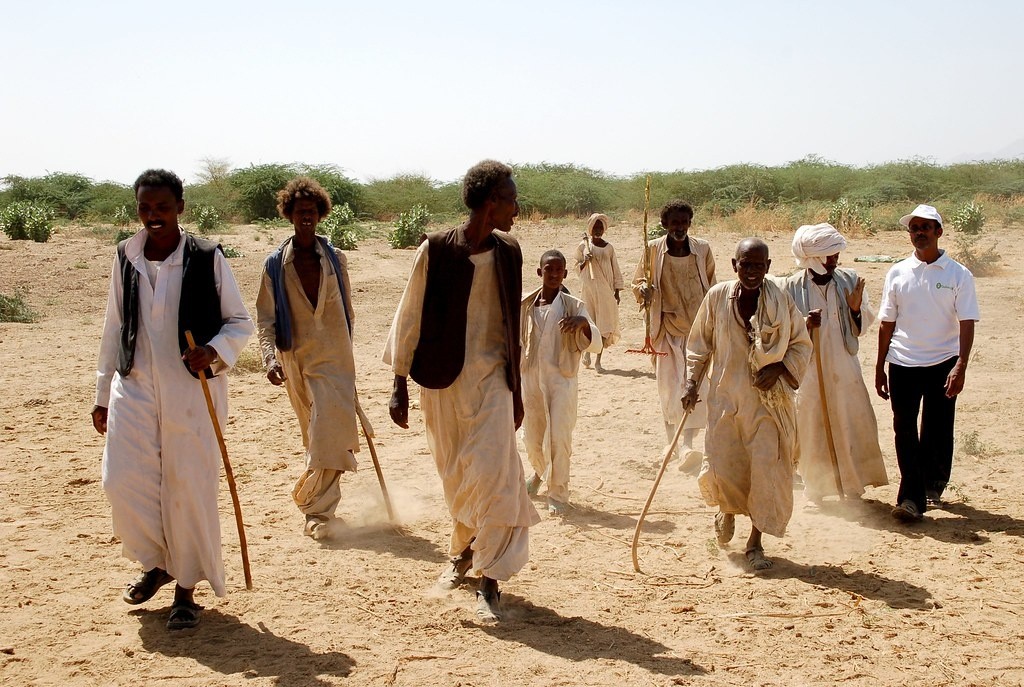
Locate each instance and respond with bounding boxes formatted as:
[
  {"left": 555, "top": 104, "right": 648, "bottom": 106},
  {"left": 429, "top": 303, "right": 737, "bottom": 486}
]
[{"left": 907, "top": 224, "right": 937, "bottom": 232}]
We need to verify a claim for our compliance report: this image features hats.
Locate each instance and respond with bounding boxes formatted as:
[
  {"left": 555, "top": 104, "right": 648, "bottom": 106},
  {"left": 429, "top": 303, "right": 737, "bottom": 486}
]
[
  {"left": 792, "top": 223, "right": 846, "bottom": 275},
  {"left": 899, "top": 203, "right": 943, "bottom": 227},
  {"left": 588, "top": 213, "right": 609, "bottom": 236}
]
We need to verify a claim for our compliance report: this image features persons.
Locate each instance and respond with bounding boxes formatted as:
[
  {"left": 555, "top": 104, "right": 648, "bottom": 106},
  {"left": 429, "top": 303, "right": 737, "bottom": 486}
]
[
  {"left": 782, "top": 223, "right": 889, "bottom": 514},
  {"left": 382, "top": 160, "right": 540, "bottom": 622},
  {"left": 256, "top": 178, "right": 360, "bottom": 536},
  {"left": 681, "top": 238, "right": 813, "bottom": 568},
  {"left": 91, "top": 169, "right": 253, "bottom": 630},
  {"left": 573, "top": 213, "right": 624, "bottom": 373},
  {"left": 631, "top": 199, "right": 717, "bottom": 471},
  {"left": 518, "top": 251, "right": 602, "bottom": 516},
  {"left": 875, "top": 204, "right": 979, "bottom": 521}
]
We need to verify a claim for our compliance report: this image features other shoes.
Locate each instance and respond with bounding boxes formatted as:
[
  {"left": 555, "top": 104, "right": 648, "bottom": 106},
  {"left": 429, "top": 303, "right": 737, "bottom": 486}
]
[
  {"left": 595, "top": 364, "right": 604, "bottom": 374},
  {"left": 792, "top": 471, "right": 803, "bottom": 489},
  {"left": 582, "top": 351, "right": 591, "bottom": 366}
]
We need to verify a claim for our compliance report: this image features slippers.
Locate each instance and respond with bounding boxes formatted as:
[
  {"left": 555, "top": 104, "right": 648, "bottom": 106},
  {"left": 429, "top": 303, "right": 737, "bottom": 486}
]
[
  {"left": 122, "top": 575, "right": 175, "bottom": 605},
  {"left": 661, "top": 449, "right": 679, "bottom": 462},
  {"left": 803, "top": 496, "right": 822, "bottom": 514},
  {"left": 304, "top": 514, "right": 338, "bottom": 536},
  {"left": 525, "top": 479, "right": 543, "bottom": 499},
  {"left": 547, "top": 497, "right": 566, "bottom": 520},
  {"left": 167, "top": 600, "right": 205, "bottom": 629},
  {"left": 679, "top": 449, "right": 702, "bottom": 470}
]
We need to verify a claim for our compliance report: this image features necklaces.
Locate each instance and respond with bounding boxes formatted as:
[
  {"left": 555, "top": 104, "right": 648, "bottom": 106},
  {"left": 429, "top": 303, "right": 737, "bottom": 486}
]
[{"left": 732, "top": 283, "right": 752, "bottom": 330}]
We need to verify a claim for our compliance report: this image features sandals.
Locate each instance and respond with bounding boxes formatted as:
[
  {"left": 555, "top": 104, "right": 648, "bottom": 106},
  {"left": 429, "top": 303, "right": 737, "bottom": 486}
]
[
  {"left": 924, "top": 492, "right": 942, "bottom": 511},
  {"left": 438, "top": 554, "right": 473, "bottom": 590},
  {"left": 713, "top": 512, "right": 735, "bottom": 543},
  {"left": 476, "top": 587, "right": 501, "bottom": 622},
  {"left": 745, "top": 546, "right": 772, "bottom": 570},
  {"left": 891, "top": 498, "right": 923, "bottom": 521}
]
